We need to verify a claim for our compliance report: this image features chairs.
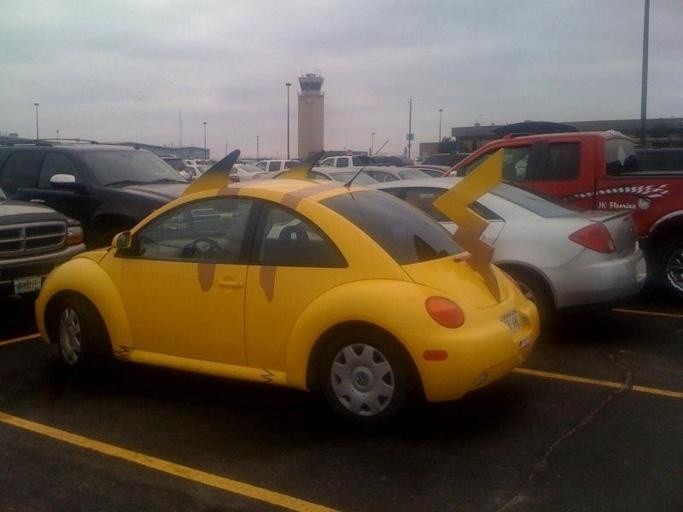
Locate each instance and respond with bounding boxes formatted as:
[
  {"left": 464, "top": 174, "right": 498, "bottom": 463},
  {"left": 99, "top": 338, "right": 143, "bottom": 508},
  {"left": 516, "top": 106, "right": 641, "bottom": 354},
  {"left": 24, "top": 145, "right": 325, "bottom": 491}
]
[{"left": 279, "top": 226, "right": 309, "bottom": 241}]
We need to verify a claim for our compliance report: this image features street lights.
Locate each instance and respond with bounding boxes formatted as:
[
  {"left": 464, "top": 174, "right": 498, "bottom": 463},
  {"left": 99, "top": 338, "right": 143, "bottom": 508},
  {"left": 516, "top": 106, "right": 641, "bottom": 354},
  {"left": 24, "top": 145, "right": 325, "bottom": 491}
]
[
  {"left": 437, "top": 108, "right": 443, "bottom": 142},
  {"left": 284, "top": 82, "right": 293, "bottom": 160},
  {"left": 203, "top": 122, "right": 207, "bottom": 159}
]
